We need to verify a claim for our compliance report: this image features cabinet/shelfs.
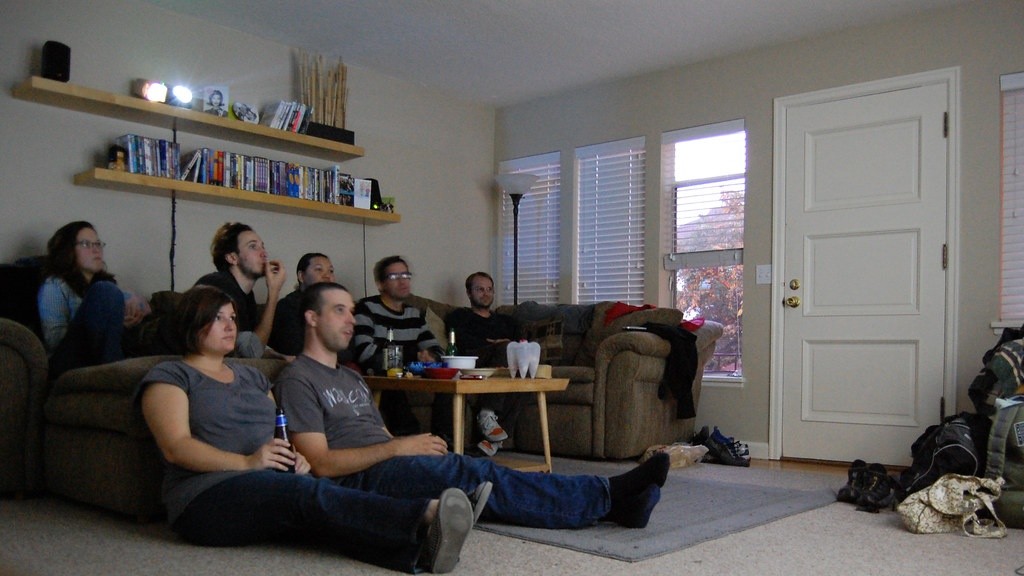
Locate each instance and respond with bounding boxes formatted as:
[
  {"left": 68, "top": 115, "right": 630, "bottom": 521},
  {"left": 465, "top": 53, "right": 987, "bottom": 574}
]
[{"left": 12, "top": 75, "right": 401, "bottom": 227}]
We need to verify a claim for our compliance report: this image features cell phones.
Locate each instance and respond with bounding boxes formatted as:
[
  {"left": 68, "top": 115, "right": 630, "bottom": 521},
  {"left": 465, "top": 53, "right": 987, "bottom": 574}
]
[
  {"left": 622, "top": 325, "right": 647, "bottom": 331},
  {"left": 460, "top": 375, "right": 486, "bottom": 380}
]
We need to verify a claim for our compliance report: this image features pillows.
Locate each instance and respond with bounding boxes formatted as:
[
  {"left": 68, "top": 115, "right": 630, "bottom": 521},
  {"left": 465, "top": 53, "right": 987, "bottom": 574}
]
[
  {"left": 511, "top": 300, "right": 595, "bottom": 367},
  {"left": 522, "top": 316, "right": 567, "bottom": 362},
  {"left": 150, "top": 290, "right": 182, "bottom": 346},
  {"left": 570, "top": 302, "right": 682, "bottom": 368}
]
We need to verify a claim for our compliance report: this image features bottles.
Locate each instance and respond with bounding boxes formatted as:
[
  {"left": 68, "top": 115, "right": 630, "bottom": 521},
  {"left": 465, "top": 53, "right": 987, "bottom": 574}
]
[
  {"left": 273, "top": 407, "right": 296, "bottom": 474},
  {"left": 446, "top": 328, "right": 458, "bottom": 356},
  {"left": 382, "top": 326, "right": 395, "bottom": 377}
]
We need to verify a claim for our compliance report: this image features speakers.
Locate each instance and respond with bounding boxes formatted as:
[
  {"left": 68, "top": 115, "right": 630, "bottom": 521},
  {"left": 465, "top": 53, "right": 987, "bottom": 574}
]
[{"left": 41, "top": 41, "right": 70, "bottom": 82}]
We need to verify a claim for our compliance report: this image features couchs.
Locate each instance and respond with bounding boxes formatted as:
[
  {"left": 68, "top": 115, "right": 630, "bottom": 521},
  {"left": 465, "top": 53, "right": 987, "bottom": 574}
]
[
  {"left": 0, "top": 253, "right": 434, "bottom": 527},
  {"left": 421, "top": 296, "right": 724, "bottom": 462}
]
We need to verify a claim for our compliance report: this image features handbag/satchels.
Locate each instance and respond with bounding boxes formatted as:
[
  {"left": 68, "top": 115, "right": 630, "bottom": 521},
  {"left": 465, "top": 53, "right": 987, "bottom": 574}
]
[{"left": 892, "top": 327, "right": 1024, "bottom": 538}]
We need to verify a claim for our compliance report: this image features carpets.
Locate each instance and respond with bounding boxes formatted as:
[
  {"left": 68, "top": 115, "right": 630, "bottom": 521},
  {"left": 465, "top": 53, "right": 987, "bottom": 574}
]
[{"left": 470, "top": 455, "right": 840, "bottom": 564}]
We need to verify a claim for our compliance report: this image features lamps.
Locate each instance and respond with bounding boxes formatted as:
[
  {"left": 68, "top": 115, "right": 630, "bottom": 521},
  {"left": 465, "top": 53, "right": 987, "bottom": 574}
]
[{"left": 494, "top": 172, "right": 540, "bottom": 306}]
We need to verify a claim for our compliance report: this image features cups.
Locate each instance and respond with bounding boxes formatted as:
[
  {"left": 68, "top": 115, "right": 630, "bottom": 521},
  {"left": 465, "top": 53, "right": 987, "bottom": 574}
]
[{"left": 387, "top": 345, "right": 403, "bottom": 377}]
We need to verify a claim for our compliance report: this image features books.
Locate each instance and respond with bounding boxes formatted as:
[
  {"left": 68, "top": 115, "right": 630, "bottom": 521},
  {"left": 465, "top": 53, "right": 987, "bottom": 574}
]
[
  {"left": 260, "top": 98, "right": 314, "bottom": 134},
  {"left": 115, "top": 134, "right": 373, "bottom": 212}
]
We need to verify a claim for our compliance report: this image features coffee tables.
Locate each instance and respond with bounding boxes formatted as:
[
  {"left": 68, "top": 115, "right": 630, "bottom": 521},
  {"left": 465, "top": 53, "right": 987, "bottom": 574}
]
[{"left": 362, "top": 374, "right": 570, "bottom": 474}]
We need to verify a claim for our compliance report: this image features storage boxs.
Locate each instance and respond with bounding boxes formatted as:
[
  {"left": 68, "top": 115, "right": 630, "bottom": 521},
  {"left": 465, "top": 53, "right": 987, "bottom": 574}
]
[{"left": 306, "top": 121, "right": 356, "bottom": 145}]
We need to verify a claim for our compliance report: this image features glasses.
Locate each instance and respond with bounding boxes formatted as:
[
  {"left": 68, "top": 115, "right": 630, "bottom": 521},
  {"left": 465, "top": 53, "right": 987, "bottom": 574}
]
[
  {"left": 77, "top": 240, "right": 106, "bottom": 250},
  {"left": 378, "top": 272, "right": 412, "bottom": 280},
  {"left": 469, "top": 287, "right": 493, "bottom": 292}
]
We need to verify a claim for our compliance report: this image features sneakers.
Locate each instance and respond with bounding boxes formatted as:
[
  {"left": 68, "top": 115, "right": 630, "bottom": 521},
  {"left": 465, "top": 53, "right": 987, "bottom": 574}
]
[
  {"left": 465, "top": 481, "right": 492, "bottom": 525},
  {"left": 837, "top": 458, "right": 896, "bottom": 509},
  {"left": 479, "top": 412, "right": 509, "bottom": 441},
  {"left": 691, "top": 425, "right": 727, "bottom": 464},
  {"left": 425, "top": 488, "right": 474, "bottom": 573},
  {"left": 478, "top": 440, "right": 503, "bottom": 457},
  {"left": 707, "top": 427, "right": 750, "bottom": 468}
]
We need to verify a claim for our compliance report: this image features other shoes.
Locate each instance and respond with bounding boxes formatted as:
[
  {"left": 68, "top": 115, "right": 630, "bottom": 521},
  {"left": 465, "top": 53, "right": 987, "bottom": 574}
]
[
  {"left": 610, "top": 452, "right": 670, "bottom": 494},
  {"left": 611, "top": 485, "right": 661, "bottom": 528}
]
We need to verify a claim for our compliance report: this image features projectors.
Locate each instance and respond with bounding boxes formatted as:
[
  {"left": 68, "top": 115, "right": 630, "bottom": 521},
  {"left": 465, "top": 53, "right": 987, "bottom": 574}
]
[{"left": 134, "top": 78, "right": 193, "bottom": 109}]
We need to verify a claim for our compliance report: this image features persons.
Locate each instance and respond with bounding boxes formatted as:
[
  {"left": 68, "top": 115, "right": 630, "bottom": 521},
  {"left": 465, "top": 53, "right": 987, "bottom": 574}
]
[
  {"left": 272, "top": 280, "right": 670, "bottom": 530},
  {"left": 337, "top": 256, "right": 454, "bottom": 452},
  {"left": 37, "top": 221, "right": 152, "bottom": 392},
  {"left": 446, "top": 272, "right": 517, "bottom": 457},
  {"left": 135, "top": 285, "right": 493, "bottom": 575},
  {"left": 182, "top": 221, "right": 287, "bottom": 359},
  {"left": 267, "top": 253, "right": 356, "bottom": 366},
  {"left": 204, "top": 90, "right": 228, "bottom": 117},
  {"left": 237, "top": 103, "right": 258, "bottom": 123}
]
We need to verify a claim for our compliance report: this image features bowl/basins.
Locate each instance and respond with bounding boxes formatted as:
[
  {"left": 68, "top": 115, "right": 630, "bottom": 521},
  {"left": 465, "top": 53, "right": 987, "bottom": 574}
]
[
  {"left": 441, "top": 356, "right": 479, "bottom": 376},
  {"left": 487, "top": 368, "right": 518, "bottom": 376},
  {"left": 407, "top": 362, "right": 448, "bottom": 378},
  {"left": 424, "top": 367, "right": 460, "bottom": 379},
  {"left": 459, "top": 368, "right": 498, "bottom": 379}
]
